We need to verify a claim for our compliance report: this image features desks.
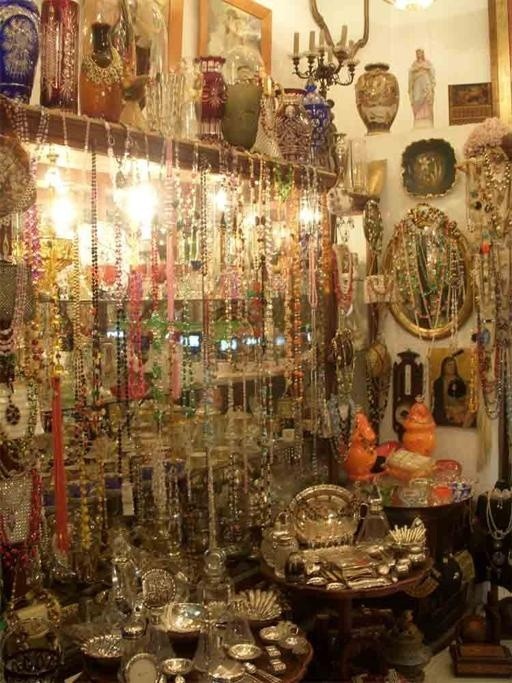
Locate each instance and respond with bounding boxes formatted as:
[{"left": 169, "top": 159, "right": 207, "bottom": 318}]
[{"left": 71, "top": 462, "right": 512, "bottom": 682}]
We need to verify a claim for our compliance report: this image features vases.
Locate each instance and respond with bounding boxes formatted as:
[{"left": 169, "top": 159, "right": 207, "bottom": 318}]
[{"left": 0, "top": 0, "right": 400, "bottom": 193}]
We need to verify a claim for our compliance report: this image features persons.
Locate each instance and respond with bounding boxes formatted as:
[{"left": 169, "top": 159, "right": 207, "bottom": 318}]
[
  {"left": 407, "top": 49, "right": 438, "bottom": 129},
  {"left": 432, "top": 357, "right": 469, "bottom": 426}
]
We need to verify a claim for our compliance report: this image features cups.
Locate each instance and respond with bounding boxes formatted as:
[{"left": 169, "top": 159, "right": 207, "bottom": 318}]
[{"left": 45, "top": 247, "right": 458, "bottom": 683}]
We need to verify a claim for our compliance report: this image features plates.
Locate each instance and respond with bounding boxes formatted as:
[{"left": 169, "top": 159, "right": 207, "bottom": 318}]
[{"left": 403, "top": 135, "right": 457, "bottom": 199}]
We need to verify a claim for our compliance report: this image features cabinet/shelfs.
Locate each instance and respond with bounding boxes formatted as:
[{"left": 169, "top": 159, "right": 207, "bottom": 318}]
[{"left": 0, "top": 122, "right": 374, "bottom": 658}]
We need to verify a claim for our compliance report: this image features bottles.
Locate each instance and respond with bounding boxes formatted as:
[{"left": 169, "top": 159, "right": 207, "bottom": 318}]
[{"left": 1, "top": 0, "right": 371, "bottom": 194}]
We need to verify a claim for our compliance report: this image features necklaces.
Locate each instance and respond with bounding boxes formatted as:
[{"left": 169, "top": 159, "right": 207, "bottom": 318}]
[{"left": 485, "top": 487, "right": 512, "bottom": 541}]
